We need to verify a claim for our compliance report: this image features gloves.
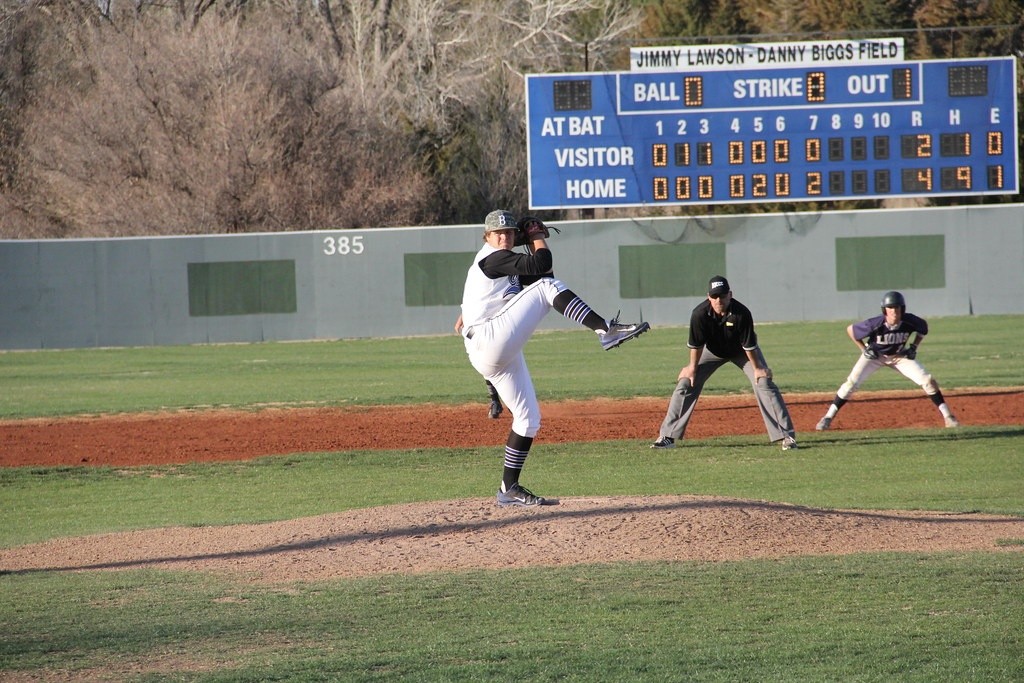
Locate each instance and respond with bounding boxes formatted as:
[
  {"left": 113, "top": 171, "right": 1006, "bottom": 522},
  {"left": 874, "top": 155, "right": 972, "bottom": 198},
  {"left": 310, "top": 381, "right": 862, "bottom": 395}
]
[
  {"left": 862, "top": 347, "right": 878, "bottom": 359},
  {"left": 906, "top": 344, "right": 917, "bottom": 360}
]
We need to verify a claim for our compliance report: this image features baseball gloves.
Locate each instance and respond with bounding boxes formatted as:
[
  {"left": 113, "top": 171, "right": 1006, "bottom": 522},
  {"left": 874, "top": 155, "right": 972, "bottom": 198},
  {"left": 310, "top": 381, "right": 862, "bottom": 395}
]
[{"left": 514, "top": 215, "right": 562, "bottom": 244}]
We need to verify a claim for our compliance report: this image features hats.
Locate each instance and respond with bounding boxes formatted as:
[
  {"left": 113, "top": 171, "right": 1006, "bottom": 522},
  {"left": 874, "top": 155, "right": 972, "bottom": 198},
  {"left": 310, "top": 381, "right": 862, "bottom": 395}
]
[
  {"left": 485, "top": 209, "right": 519, "bottom": 231},
  {"left": 881, "top": 291, "right": 905, "bottom": 315},
  {"left": 708, "top": 275, "right": 730, "bottom": 295}
]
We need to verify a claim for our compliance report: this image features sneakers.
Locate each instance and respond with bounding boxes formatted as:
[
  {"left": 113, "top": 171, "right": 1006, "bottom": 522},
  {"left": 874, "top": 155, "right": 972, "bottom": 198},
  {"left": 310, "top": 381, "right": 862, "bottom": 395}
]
[
  {"left": 594, "top": 318, "right": 650, "bottom": 351},
  {"left": 945, "top": 415, "right": 959, "bottom": 428},
  {"left": 488, "top": 401, "right": 503, "bottom": 418},
  {"left": 782, "top": 436, "right": 797, "bottom": 451},
  {"left": 816, "top": 417, "right": 832, "bottom": 430},
  {"left": 649, "top": 436, "right": 675, "bottom": 449},
  {"left": 497, "top": 482, "right": 545, "bottom": 508}
]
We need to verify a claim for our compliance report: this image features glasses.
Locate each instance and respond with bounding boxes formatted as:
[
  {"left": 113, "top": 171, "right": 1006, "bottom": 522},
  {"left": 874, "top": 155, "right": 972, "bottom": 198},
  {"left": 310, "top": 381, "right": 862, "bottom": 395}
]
[
  {"left": 710, "top": 294, "right": 727, "bottom": 299},
  {"left": 494, "top": 230, "right": 514, "bottom": 236}
]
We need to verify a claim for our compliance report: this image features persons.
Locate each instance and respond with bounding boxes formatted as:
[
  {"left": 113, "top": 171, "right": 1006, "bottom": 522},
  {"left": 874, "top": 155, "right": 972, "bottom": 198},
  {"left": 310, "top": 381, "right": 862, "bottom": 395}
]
[
  {"left": 649, "top": 275, "right": 797, "bottom": 450},
  {"left": 456, "top": 316, "right": 512, "bottom": 418},
  {"left": 461, "top": 210, "right": 652, "bottom": 508},
  {"left": 816, "top": 291, "right": 960, "bottom": 430}
]
